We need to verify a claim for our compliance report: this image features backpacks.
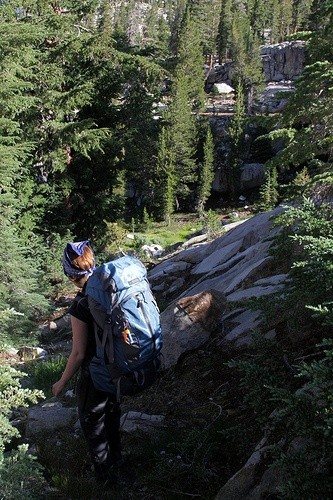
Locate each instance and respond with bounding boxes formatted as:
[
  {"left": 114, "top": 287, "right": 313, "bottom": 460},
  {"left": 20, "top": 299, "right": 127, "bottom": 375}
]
[{"left": 72, "top": 254, "right": 166, "bottom": 405}]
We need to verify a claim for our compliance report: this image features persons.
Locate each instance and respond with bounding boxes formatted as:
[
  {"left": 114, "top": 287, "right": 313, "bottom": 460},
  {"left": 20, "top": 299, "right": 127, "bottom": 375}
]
[{"left": 51, "top": 240, "right": 135, "bottom": 500}]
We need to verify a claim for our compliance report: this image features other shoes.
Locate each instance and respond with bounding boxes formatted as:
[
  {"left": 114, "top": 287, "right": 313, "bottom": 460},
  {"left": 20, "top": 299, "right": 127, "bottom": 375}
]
[{"left": 87, "top": 477, "right": 116, "bottom": 490}]
